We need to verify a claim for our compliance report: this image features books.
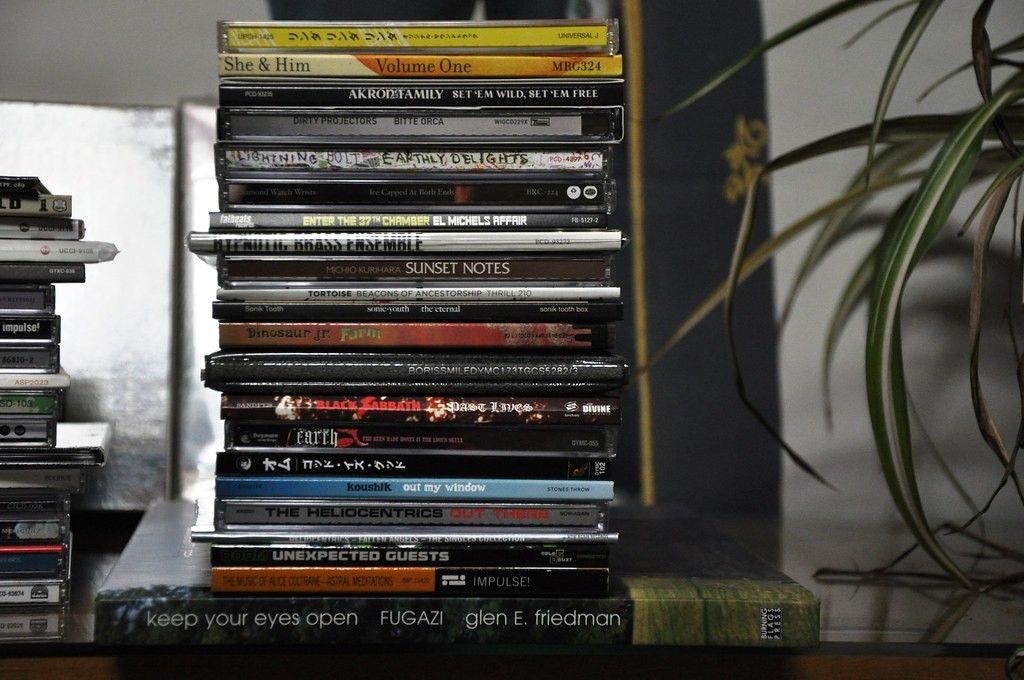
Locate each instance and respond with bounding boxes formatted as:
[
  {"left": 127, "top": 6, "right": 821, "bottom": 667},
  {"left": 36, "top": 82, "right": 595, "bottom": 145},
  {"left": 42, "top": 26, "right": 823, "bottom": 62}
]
[{"left": 1, "top": 20, "right": 820, "bottom": 651}]
